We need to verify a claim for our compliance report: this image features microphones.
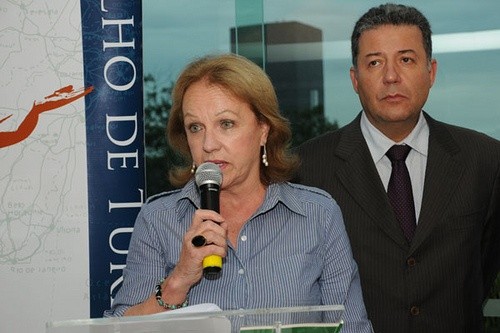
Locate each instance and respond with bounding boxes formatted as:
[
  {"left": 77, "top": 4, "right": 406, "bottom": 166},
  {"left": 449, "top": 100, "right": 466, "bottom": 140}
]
[{"left": 195, "top": 162, "right": 223, "bottom": 274}]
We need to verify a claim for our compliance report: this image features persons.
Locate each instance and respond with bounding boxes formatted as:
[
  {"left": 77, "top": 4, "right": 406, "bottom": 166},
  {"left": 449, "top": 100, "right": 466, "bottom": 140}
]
[
  {"left": 282, "top": 3, "right": 500, "bottom": 333},
  {"left": 102, "top": 53, "right": 374, "bottom": 332}
]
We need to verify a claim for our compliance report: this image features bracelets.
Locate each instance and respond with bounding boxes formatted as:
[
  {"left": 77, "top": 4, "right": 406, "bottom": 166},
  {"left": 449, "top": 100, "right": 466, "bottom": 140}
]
[{"left": 155, "top": 277, "right": 189, "bottom": 311}]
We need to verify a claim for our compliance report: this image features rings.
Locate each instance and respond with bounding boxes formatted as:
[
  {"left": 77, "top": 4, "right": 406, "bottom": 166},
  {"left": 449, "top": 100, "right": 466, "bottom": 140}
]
[{"left": 190, "top": 234, "right": 208, "bottom": 247}]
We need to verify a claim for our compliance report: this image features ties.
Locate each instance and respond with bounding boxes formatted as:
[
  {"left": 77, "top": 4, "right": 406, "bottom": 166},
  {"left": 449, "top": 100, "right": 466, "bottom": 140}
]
[{"left": 385, "top": 143, "right": 418, "bottom": 244}]
